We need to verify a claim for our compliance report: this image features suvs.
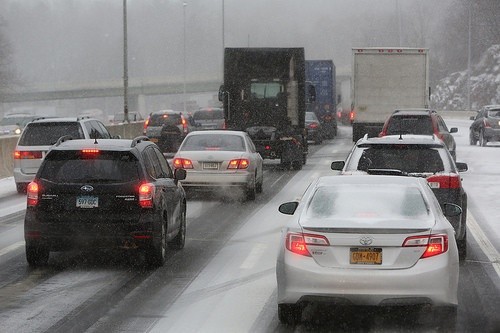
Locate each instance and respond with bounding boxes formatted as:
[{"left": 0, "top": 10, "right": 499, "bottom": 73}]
[
  {"left": 468, "top": 105, "right": 500, "bottom": 147},
  {"left": 23, "top": 132, "right": 187, "bottom": 267},
  {"left": 12, "top": 116, "right": 121, "bottom": 195},
  {"left": 378, "top": 109, "right": 458, "bottom": 162},
  {"left": 142, "top": 110, "right": 200, "bottom": 148},
  {"left": 330, "top": 133, "right": 468, "bottom": 241}
]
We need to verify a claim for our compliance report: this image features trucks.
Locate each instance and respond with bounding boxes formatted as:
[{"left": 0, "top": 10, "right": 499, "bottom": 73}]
[
  {"left": 217, "top": 45, "right": 316, "bottom": 171},
  {"left": 348, "top": 45, "right": 432, "bottom": 143},
  {"left": 305, "top": 59, "right": 341, "bottom": 136}
]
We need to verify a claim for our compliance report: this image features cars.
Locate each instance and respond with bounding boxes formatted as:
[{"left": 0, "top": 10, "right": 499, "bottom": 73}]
[
  {"left": 304, "top": 111, "right": 324, "bottom": 145},
  {"left": 0, "top": 107, "right": 144, "bottom": 135},
  {"left": 172, "top": 129, "right": 264, "bottom": 200},
  {"left": 337, "top": 103, "right": 351, "bottom": 125},
  {"left": 274, "top": 173, "right": 463, "bottom": 326},
  {"left": 192, "top": 107, "right": 226, "bottom": 131}
]
